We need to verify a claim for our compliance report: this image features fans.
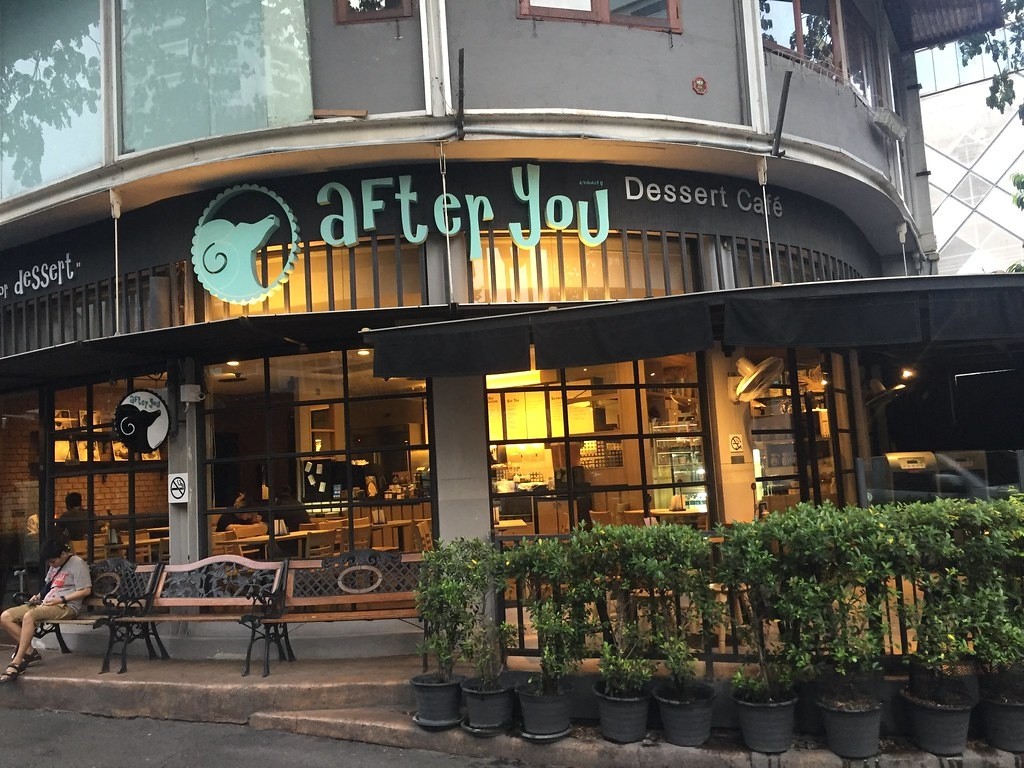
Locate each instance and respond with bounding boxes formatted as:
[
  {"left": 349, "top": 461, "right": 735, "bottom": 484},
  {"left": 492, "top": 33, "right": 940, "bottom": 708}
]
[{"left": 732, "top": 345, "right": 784, "bottom": 404}]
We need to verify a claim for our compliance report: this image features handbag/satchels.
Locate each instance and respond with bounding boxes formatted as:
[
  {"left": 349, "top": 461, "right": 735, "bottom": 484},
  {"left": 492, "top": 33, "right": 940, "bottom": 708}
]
[{"left": 40, "top": 580, "right": 55, "bottom": 600}]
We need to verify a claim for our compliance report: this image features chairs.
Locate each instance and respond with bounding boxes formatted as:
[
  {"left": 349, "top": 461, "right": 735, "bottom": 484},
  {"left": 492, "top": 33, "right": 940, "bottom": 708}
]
[{"left": 31, "top": 496, "right": 627, "bottom": 561}]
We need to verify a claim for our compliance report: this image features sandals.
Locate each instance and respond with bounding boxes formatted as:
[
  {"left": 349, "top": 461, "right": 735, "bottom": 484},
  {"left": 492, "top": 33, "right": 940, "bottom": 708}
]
[
  {"left": 23, "top": 648, "right": 41, "bottom": 667},
  {"left": 0, "top": 663, "right": 26, "bottom": 683}
]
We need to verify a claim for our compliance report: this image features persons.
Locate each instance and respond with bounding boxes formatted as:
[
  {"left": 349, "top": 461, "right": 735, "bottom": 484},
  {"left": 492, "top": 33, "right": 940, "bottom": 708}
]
[
  {"left": 0, "top": 541, "right": 92, "bottom": 684},
  {"left": 214, "top": 487, "right": 312, "bottom": 561},
  {"left": 27, "top": 492, "right": 100, "bottom": 541}
]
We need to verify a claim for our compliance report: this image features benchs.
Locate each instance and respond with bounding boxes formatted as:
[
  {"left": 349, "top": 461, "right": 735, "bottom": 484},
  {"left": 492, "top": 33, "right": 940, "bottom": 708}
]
[
  {"left": 12, "top": 558, "right": 163, "bottom": 677},
  {"left": 253, "top": 548, "right": 444, "bottom": 678},
  {"left": 110, "top": 552, "right": 287, "bottom": 679}
]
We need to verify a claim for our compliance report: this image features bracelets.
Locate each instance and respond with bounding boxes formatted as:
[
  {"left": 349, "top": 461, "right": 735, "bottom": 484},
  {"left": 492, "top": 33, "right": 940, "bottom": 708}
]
[{"left": 60, "top": 596, "right": 66, "bottom": 602}]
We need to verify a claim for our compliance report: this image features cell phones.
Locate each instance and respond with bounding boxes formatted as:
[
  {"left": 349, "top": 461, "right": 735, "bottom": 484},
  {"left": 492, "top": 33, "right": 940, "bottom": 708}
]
[{"left": 24, "top": 600, "right": 43, "bottom": 604}]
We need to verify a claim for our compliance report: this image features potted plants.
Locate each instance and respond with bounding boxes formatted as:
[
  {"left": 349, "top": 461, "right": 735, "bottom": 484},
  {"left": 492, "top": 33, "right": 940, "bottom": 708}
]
[{"left": 407, "top": 485, "right": 1024, "bottom": 757}]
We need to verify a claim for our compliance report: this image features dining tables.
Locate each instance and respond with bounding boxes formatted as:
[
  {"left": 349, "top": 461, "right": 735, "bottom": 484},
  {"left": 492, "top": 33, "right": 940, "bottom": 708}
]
[{"left": 622, "top": 503, "right": 711, "bottom": 530}]
